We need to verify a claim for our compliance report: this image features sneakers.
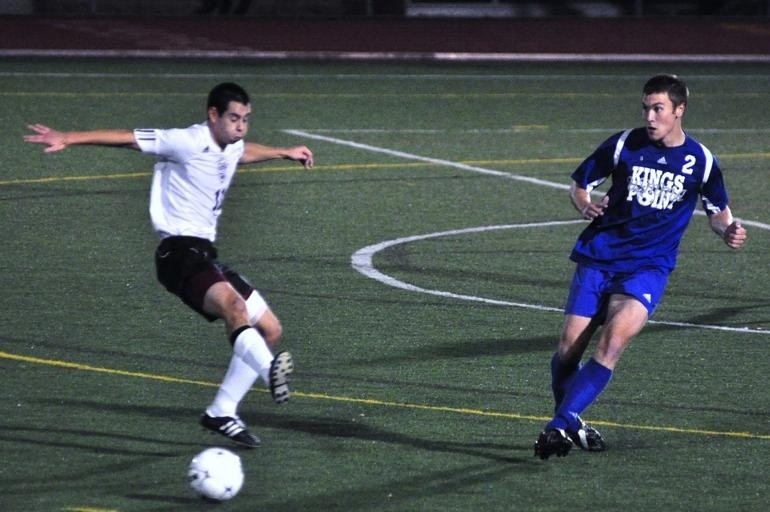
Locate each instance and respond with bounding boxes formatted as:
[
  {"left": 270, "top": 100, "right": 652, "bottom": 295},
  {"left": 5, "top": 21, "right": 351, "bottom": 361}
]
[
  {"left": 198, "top": 411, "right": 261, "bottom": 446},
  {"left": 535, "top": 429, "right": 571, "bottom": 460},
  {"left": 568, "top": 425, "right": 605, "bottom": 450},
  {"left": 271, "top": 350, "right": 297, "bottom": 404}
]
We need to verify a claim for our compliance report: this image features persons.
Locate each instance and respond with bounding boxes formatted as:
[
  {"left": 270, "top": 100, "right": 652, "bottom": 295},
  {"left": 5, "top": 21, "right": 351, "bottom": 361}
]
[
  {"left": 533, "top": 75, "right": 748, "bottom": 460},
  {"left": 22, "top": 82, "right": 314, "bottom": 449}
]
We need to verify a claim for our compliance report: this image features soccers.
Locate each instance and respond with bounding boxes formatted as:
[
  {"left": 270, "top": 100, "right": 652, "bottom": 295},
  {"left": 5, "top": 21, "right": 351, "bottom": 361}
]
[{"left": 187, "top": 447, "right": 244, "bottom": 501}]
